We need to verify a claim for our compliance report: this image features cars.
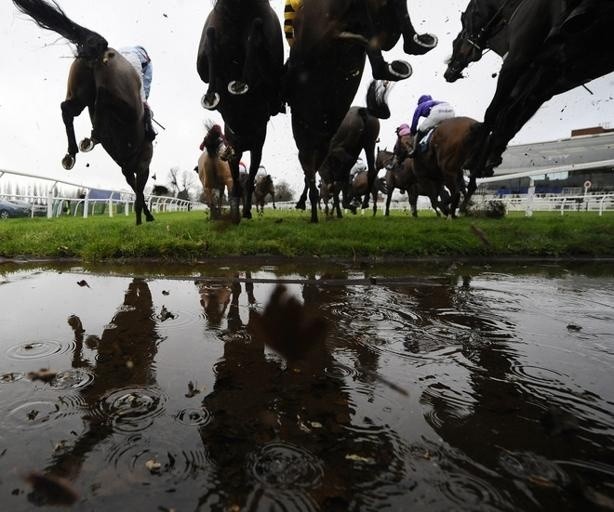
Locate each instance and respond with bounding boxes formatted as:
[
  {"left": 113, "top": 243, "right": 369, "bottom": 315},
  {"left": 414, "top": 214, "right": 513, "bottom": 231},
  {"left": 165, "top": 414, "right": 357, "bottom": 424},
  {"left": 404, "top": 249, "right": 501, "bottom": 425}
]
[{"left": 0, "top": 200, "right": 48, "bottom": 217}]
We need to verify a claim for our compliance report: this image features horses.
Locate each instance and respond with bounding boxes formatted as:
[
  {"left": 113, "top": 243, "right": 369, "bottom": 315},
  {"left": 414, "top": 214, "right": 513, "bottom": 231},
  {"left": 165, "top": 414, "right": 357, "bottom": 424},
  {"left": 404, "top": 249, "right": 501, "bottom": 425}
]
[
  {"left": 443, "top": 0, "right": 614, "bottom": 179},
  {"left": 11, "top": 0, "right": 156, "bottom": 227},
  {"left": 281, "top": 0, "right": 439, "bottom": 224},
  {"left": 315, "top": 79, "right": 492, "bottom": 227},
  {"left": 194, "top": 123, "right": 277, "bottom": 220},
  {"left": 195, "top": 0, "right": 285, "bottom": 226}
]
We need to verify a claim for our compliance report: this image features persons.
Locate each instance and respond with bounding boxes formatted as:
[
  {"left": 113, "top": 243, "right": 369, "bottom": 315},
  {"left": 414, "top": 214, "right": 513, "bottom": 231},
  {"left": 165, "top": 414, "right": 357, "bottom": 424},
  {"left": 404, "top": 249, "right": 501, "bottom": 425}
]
[
  {"left": 349, "top": 158, "right": 366, "bottom": 183},
  {"left": 200, "top": 125, "right": 229, "bottom": 156},
  {"left": 239, "top": 162, "right": 247, "bottom": 174},
  {"left": 284, "top": 0, "right": 303, "bottom": 47},
  {"left": 254, "top": 166, "right": 267, "bottom": 185},
  {"left": 395, "top": 124, "right": 412, "bottom": 158},
  {"left": 410, "top": 94, "right": 455, "bottom": 155},
  {"left": 121, "top": 46, "right": 152, "bottom": 105}
]
